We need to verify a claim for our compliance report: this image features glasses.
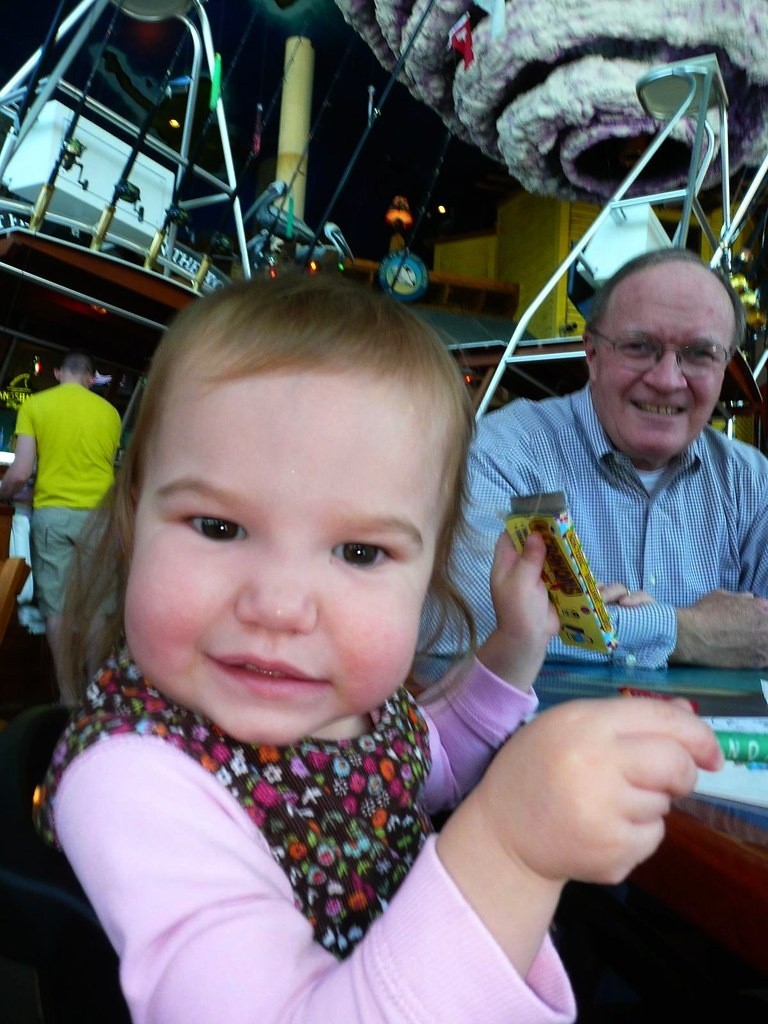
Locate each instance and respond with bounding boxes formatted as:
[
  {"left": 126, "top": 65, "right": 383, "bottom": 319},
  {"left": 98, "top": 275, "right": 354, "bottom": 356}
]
[{"left": 590, "top": 327, "right": 733, "bottom": 378}]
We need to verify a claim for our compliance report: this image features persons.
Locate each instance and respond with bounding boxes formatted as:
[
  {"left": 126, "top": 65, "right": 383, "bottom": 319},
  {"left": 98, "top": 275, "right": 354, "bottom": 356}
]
[
  {"left": 29, "top": 273, "right": 724, "bottom": 1024},
  {"left": 0, "top": 351, "right": 122, "bottom": 710},
  {"left": 414, "top": 248, "right": 768, "bottom": 670}
]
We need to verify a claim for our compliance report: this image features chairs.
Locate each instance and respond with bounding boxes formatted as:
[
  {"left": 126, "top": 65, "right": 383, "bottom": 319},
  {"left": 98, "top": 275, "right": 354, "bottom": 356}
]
[{"left": 0, "top": 704, "right": 134, "bottom": 1024}]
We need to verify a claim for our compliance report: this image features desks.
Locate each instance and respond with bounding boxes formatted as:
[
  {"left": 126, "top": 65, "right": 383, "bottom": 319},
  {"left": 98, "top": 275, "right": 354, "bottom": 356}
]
[{"left": 403, "top": 658, "right": 768, "bottom": 978}]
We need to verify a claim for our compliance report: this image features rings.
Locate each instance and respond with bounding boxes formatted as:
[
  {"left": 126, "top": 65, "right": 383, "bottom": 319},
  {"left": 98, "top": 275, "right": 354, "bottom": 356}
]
[{"left": 626, "top": 588, "right": 631, "bottom": 596}]
